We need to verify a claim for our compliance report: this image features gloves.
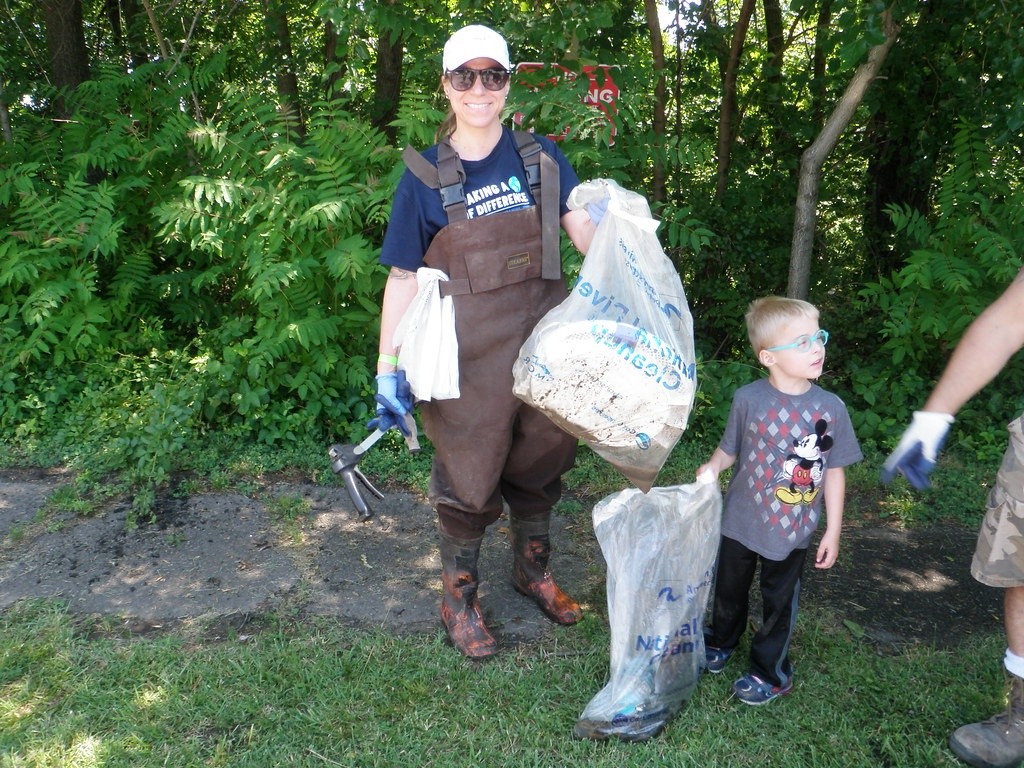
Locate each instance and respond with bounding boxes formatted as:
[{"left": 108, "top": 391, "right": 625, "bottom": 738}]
[
  {"left": 882, "top": 412, "right": 956, "bottom": 490},
  {"left": 363, "top": 370, "right": 415, "bottom": 437}
]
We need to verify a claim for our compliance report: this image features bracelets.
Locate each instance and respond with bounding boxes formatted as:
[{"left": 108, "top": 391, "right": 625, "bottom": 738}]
[{"left": 378, "top": 354, "right": 398, "bottom": 365}]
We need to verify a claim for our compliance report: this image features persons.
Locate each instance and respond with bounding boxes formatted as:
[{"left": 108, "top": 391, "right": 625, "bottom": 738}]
[
  {"left": 697, "top": 297, "right": 863, "bottom": 706},
  {"left": 372, "top": 24, "right": 608, "bottom": 660},
  {"left": 879, "top": 271, "right": 1024, "bottom": 768}
]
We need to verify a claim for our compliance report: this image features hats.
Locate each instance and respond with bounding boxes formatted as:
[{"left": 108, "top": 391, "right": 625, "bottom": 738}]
[{"left": 442, "top": 24, "right": 509, "bottom": 75}]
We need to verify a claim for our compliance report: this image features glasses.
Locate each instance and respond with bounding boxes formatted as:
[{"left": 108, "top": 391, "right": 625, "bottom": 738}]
[
  {"left": 446, "top": 68, "right": 509, "bottom": 92},
  {"left": 766, "top": 330, "right": 829, "bottom": 352}
]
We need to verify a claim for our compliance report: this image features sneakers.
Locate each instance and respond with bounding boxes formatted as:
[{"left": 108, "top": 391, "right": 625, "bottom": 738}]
[
  {"left": 732, "top": 672, "right": 793, "bottom": 705},
  {"left": 703, "top": 645, "right": 735, "bottom": 673}
]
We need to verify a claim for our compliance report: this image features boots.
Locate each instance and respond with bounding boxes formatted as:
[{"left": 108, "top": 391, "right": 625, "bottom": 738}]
[
  {"left": 509, "top": 510, "right": 584, "bottom": 625},
  {"left": 949, "top": 648, "right": 1023, "bottom": 768},
  {"left": 438, "top": 521, "right": 498, "bottom": 660}
]
